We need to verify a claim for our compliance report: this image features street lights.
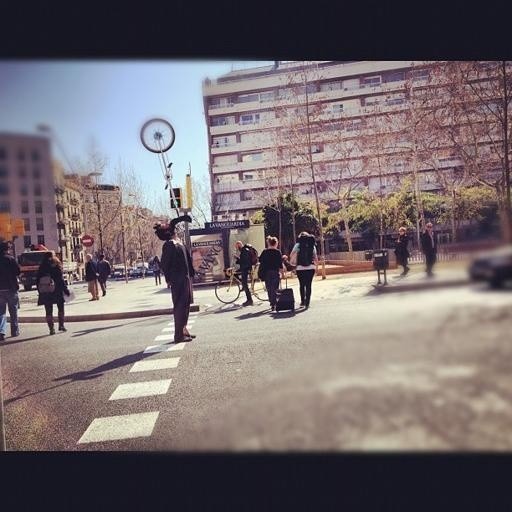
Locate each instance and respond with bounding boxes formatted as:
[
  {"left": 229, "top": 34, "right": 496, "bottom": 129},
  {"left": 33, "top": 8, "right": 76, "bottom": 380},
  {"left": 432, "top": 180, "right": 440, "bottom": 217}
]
[{"left": 83, "top": 172, "right": 102, "bottom": 235}]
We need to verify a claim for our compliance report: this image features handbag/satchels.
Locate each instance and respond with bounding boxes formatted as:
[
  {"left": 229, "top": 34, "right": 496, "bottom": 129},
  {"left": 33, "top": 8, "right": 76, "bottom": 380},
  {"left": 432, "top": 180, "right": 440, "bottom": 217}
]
[{"left": 257, "top": 249, "right": 267, "bottom": 281}]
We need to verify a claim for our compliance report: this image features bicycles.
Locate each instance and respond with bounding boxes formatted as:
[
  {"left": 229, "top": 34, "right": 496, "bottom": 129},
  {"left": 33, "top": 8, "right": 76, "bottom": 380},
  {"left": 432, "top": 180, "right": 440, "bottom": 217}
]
[{"left": 214, "top": 266, "right": 279, "bottom": 303}]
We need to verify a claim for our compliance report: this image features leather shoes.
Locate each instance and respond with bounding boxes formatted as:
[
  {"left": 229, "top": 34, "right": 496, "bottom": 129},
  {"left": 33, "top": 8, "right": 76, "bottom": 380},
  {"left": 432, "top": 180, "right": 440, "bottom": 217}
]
[
  {"left": 175, "top": 337, "right": 192, "bottom": 343},
  {"left": 58, "top": 327, "right": 67, "bottom": 331},
  {"left": 186, "top": 335, "right": 196, "bottom": 339}
]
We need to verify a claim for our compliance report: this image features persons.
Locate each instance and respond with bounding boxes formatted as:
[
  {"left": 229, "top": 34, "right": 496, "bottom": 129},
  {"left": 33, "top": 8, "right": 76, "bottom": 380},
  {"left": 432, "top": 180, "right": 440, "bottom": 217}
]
[
  {"left": 152, "top": 256, "right": 161, "bottom": 285},
  {"left": 421, "top": 222, "right": 438, "bottom": 276},
  {"left": 161, "top": 229, "right": 197, "bottom": 344},
  {"left": 36, "top": 251, "right": 70, "bottom": 335},
  {"left": 259, "top": 235, "right": 287, "bottom": 312},
  {"left": 85, "top": 254, "right": 99, "bottom": 301},
  {"left": 281, "top": 255, "right": 296, "bottom": 272},
  {"left": 0, "top": 241, "right": 21, "bottom": 340},
  {"left": 231, "top": 240, "right": 253, "bottom": 306},
  {"left": 290, "top": 231, "right": 319, "bottom": 310},
  {"left": 394, "top": 227, "right": 411, "bottom": 276},
  {"left": 96, "top": 255, "right": 112, "bottom": 296}
]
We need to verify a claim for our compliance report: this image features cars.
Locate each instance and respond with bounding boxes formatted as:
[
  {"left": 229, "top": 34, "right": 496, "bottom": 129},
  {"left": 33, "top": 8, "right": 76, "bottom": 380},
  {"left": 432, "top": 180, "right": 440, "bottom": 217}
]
[
  {"left": 112, "top": 266, "right": 154, "bottom": 278},
  {"left": 469, "top": 241, "right": 512, "bottom": 288}
]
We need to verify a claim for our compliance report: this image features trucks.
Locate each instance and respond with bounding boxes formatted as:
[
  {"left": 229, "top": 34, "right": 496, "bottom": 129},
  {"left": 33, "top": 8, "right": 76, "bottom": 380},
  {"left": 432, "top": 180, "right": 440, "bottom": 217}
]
[{"left": 18, "top": 244, "right": 78, "bottom": 290}]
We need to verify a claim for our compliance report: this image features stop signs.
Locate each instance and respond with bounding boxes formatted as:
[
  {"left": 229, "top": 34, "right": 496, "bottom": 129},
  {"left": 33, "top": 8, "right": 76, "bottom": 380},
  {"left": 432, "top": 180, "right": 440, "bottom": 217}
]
[{"left": 81, "top": 235, "right": 94, "bottom": 246}]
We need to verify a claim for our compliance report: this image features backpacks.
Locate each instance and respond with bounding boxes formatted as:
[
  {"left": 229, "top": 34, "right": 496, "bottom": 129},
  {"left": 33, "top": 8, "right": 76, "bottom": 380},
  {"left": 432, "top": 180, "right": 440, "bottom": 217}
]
[
  {"left": 38, "top": 276, "right": 55, "bottom": 293},
  {"left": 243, "top": 243, "right": 258, "bottom": 265},
  {"left": 298, "top": 234, "right": 315, "bottom": 266}
]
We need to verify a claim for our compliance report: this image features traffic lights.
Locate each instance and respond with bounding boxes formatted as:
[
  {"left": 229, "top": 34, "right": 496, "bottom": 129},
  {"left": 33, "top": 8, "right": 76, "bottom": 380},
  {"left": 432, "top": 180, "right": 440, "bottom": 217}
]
[{"left": 171, "top": 199, "right": 181, "bottom": 208}]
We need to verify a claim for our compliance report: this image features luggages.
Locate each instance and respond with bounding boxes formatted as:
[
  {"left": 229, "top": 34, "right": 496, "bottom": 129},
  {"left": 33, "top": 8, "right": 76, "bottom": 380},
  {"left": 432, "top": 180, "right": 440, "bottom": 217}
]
[{"left": 275, "top": 277, "right": 294, "bottom": 313}]
